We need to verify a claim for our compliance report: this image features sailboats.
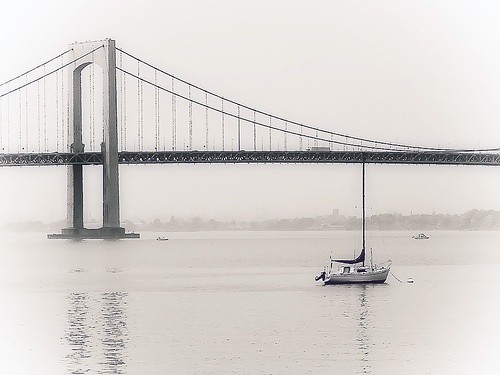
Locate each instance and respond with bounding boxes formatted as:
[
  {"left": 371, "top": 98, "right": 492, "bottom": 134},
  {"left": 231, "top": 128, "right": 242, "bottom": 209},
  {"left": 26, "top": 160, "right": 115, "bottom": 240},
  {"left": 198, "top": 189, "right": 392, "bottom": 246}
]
[{"left": 315, "top": 151, "right": 393, "bottom": 287}]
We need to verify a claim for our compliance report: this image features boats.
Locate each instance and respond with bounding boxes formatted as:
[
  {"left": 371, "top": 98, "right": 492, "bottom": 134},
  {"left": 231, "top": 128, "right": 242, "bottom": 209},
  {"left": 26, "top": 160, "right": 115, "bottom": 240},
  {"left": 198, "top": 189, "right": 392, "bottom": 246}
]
[
  {"left": 414, "top": 233, "right": 430, "bottom": 240},
  {"left": 156, "top": 235, "right": 168, "bottom": 242}
]
